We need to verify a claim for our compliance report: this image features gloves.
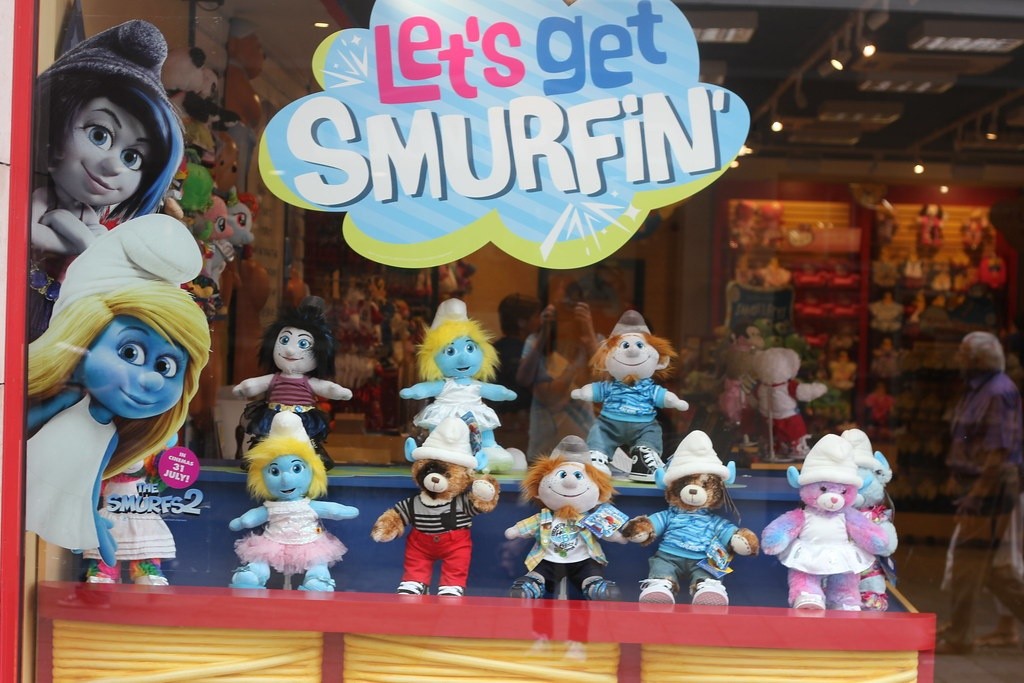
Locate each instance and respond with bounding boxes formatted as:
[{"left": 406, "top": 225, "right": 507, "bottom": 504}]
[{"left": 951, "top": 497, "right": 981, "bottom": 530}]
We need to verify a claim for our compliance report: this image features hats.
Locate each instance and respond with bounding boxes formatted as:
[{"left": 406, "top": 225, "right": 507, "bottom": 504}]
[
  {"left": 948, "top": 332, "right": 1005, "bottom": 373},
  {"left": 497, "top": 292, "right": 545, "bottom": 315}
]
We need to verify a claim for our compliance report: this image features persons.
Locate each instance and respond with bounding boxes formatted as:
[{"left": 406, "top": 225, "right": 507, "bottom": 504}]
[
  {"left": 922, "top": 332, "right": 1024, "bottom": 651},
  {"left": 489, "top": 292, "right": 545, "bottom": 409},
  {"left": 513, "top": 276, "right": 609, "bottom": 667}
]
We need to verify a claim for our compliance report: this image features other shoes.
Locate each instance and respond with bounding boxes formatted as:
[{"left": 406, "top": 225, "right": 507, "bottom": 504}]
[
  {"left": 935, "top": 638, "right": 968, "bottom": 655},
  {"left": 936, "top": 621, "right": 957, "bottom": 634},
  {"left": 977, "top": 633, "right": 1017, "bottom": 647}
]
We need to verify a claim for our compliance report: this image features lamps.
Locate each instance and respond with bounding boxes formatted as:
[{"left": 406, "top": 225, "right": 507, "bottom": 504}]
[
  {"left": 770, "top": 100, "right": 783, "bottom": 133},
  {"left": 985, "top": 108, "right": 1000, "bottom": 140},
  {"left": 913, "top": 149, "right": 924, "bottom": 174},
  {"left": 820, "top": 10, "right": 889, "bottom": 76}
]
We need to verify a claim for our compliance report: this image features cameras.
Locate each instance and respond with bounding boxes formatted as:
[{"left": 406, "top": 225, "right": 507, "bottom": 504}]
[{"left": 552, "top": 299, "right": 577, "bottom": 319}]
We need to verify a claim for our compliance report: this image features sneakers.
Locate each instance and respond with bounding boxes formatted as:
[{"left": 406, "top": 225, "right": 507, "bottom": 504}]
[
  {"left": 525, "top": 634, "right": 551, "bottom": 656},
  {"left": 564, "top": 640, "right": 586, "bottom": 661}
]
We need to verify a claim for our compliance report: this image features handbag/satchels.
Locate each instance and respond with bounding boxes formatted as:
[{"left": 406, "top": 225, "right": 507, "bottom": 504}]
[{"left": 940, "top": 465, "right": 1019, "bottom": 516}]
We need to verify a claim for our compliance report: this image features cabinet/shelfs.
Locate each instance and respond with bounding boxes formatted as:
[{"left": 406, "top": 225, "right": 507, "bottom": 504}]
[
  {"left": 862, "top": 182, "right": 1024, "bottom": 515},
  {"left": 711, "top": 182, "right": 866, "bottom": 430}
]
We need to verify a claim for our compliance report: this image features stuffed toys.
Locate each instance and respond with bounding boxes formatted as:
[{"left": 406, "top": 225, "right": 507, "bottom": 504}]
[
  {"left": 72, "top": 435, "right": 176, "bottom": 584},
  {"left": 228, "top": 411, "right": 358, "bottom": 600},
  {"left": 503, "top": 435, "right": 629, "bottom": 600},
  {"left": 621, "top": 431, "right": 759, "bottom": 606},
  {"left": 571, "top": 308, "right": 688, "bottom": 483},
  {"left": 369, "top": 417, "right": 500, "bottom": 605},
  {"left": 232, "top": 306, "right": 353, "bottom": 475},
  {"left": 399, "top": 299, "right": 517, "bottom": 473},
  {"left": 30, "top": 45, "right": 1024, "bottom": 462},
  {"left": 761, "top": 433, "right": 890, "bottom": 610}
]
